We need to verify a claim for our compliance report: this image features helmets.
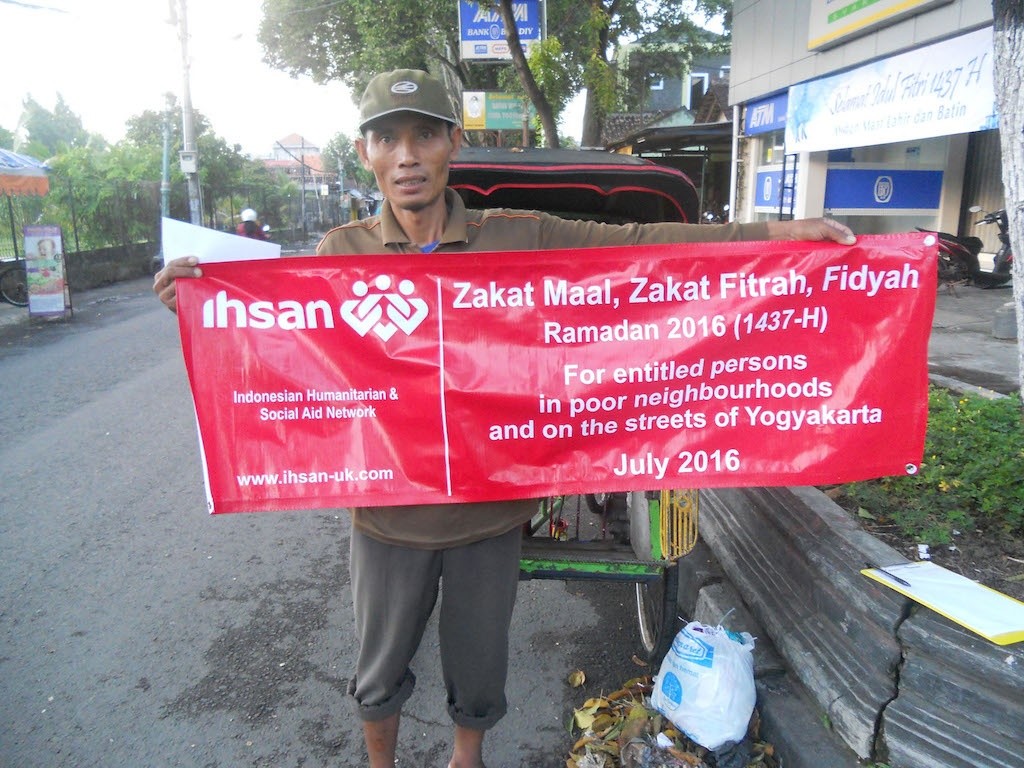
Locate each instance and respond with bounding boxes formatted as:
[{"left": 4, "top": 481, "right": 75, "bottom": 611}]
[{"left": 241, "top": 208, "right": 258, "bottom": 223}]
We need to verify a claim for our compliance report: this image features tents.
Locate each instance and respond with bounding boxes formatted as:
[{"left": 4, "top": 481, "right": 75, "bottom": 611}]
[{"left": 0, "top": 150, "right": 74, "bottom": 318}]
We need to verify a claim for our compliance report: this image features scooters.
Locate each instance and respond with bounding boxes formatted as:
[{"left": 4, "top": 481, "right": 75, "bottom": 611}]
[{"left": 911, "top": 206, "right": 1013, "bottom": 298}]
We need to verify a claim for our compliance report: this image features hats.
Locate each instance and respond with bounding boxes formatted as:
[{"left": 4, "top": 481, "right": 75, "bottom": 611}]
[{"left": 358, "top": 71, "right": 457, "bottom": 131}]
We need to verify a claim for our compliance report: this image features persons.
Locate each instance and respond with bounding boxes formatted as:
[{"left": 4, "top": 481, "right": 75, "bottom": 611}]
[
  {"left": 151, "top": 69, "right": 858, "bottom": 768},
  {"left": 26, "top": 238, "right": 56, "bottom": 259}
]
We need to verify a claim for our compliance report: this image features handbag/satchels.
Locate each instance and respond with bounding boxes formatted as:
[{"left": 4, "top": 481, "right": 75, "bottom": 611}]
[{"left": 650, "top": 622, "right": 758, "bottom": 750}]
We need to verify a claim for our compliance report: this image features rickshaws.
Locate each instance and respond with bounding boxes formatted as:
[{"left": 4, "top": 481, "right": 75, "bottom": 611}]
[{"left": 448, "top": 149, "right": 701, "bottom": 664}]
